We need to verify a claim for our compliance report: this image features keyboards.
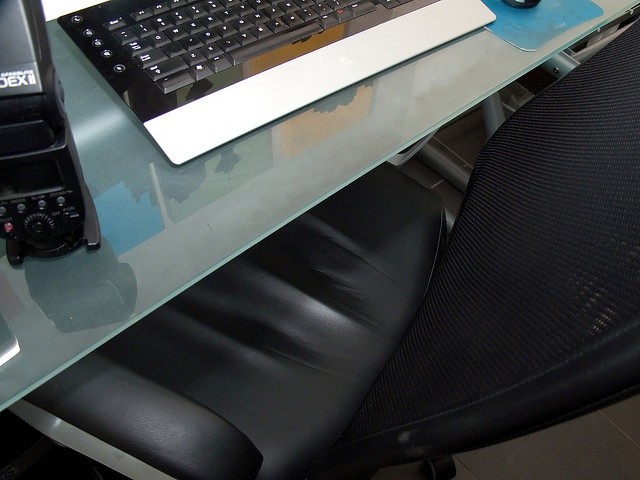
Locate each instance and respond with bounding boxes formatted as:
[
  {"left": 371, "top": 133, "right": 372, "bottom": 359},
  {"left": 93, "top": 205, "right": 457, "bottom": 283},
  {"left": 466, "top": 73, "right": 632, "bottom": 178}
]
[{"left": 56, "top": 0, "right": 496, "bottom": 168}]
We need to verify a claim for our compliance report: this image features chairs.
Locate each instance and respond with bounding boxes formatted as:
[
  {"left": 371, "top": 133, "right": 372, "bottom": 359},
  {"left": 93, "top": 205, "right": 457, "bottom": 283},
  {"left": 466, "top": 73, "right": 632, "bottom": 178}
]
[{"left": 9, "top": 22, "right": 639, "bottom": 479}]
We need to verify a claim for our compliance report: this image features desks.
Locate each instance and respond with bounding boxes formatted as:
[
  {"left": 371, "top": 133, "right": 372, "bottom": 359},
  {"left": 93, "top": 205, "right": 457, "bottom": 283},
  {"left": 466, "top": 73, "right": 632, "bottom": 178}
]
[{"left": 1, "top": 0, "right": 636, "bottom": 411}]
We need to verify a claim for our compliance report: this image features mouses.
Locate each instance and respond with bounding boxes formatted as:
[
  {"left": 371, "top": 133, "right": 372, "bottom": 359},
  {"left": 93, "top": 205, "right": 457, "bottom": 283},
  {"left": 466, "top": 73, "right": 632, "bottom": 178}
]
[{"left": 502, "top": 0, "right": 540, "bottom": 13}]
